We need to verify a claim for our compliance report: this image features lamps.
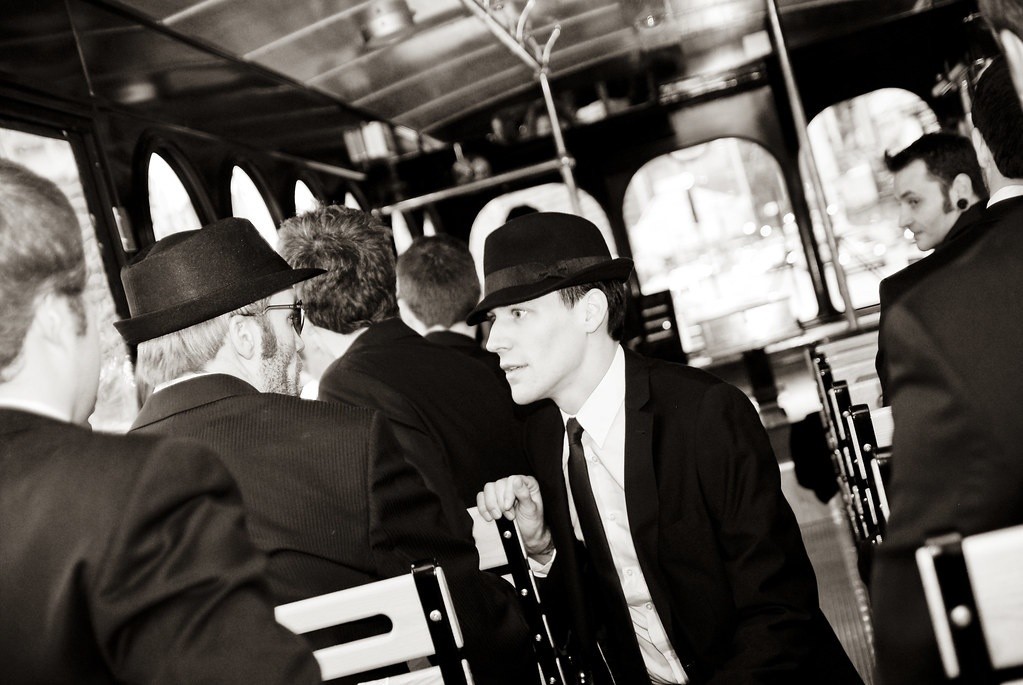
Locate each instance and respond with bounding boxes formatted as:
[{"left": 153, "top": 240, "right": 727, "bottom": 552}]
[{"left": 359, "top": 0, "right": 416, "bottom": 48}]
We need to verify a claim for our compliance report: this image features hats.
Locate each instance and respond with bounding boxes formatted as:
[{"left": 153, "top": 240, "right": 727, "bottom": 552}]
[
  {"left": 466, "top": 205, "right": 633, "bottom": 327},
  {"left": 115, "top": 217, "right": 329, "bottom": 348}
]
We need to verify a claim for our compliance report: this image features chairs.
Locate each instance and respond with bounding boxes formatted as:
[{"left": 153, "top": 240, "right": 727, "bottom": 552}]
[
  {"left": 809, "top": 328, "right": 1023, "bottom": 685},
  {"left": 270, "top": 499, "right": 617, "bottom": 685}
]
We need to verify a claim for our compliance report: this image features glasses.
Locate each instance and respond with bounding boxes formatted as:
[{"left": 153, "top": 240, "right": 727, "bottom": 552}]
[{"left": 241, "top": 299, "right": 305, "bottom": 336}]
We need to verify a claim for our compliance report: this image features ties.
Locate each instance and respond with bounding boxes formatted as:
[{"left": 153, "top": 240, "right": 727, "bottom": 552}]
[{"left": 564, "top": 418, "right": 654, "bottom": 685}]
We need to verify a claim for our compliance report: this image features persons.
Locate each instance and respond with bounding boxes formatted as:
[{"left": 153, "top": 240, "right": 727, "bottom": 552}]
[
  {"left": 283, "top": 204, "right": 594, "bottom": 683},
  {"left": 467, "top": 210, "right": 864, "bottom": 685},
  {"left": 0, "top": 156, "right": 326, "bottom": 685},
  {"left": 107, "top": 221, "right": 457, "bottom": 685},
  {"left": 862, "top": 1, "right": 1023, "bottom": 685}
]
